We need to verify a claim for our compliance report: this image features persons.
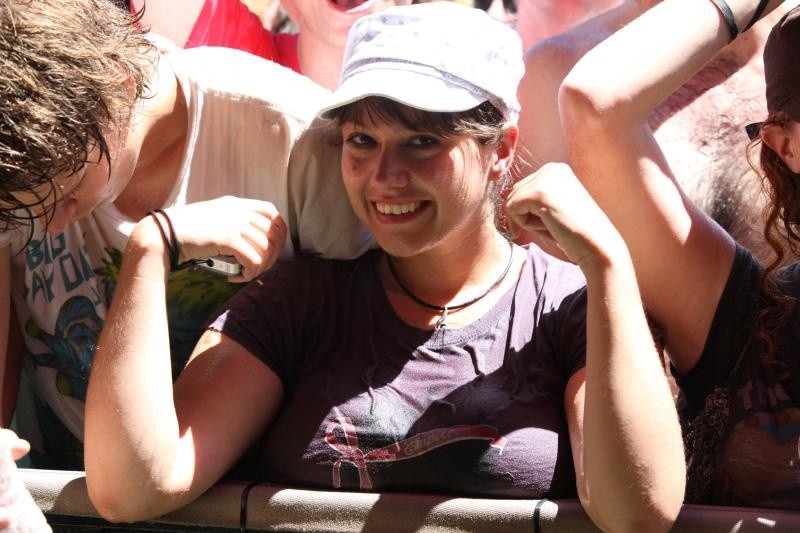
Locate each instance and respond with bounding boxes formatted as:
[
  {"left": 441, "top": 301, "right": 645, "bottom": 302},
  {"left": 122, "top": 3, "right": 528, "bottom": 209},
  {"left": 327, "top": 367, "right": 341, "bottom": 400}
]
[
  {"left": 84, "top": 0, "right": 687, "bottom": 533},
  {"left": 130, "top": 0, "right": 800, "bottom": 396},
  {"left": 0, "top": 0, "right": 377, "bottom": 531},
  {"left": 556, "top": 0, "right": 800, "bottom": 510}
]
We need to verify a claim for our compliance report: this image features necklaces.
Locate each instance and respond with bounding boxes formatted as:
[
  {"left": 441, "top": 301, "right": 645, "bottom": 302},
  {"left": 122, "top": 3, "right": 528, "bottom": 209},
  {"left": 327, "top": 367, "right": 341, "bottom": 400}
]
[{"left": 386, "top": 228, "right": 513, "bottom": 331}]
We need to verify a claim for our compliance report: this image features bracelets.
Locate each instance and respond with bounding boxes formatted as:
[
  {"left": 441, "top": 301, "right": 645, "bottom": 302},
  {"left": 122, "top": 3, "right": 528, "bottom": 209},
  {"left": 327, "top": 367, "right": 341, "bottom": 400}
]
[
  {"left": 712, "top": 0, "right": 738, "bottom": 44},
  {"left": 742, "top": 0, "right": 770, "bottom": 33}
]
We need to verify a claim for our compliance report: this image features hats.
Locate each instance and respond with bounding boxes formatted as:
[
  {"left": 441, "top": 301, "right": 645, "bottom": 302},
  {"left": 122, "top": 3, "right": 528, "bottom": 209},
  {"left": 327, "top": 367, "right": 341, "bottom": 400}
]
[
  {"left": 744, "top": 4, "right": 800, "bottom": 133},
  {"left": 319, "top": 3, "right": 524, "bottom": 128}
]
